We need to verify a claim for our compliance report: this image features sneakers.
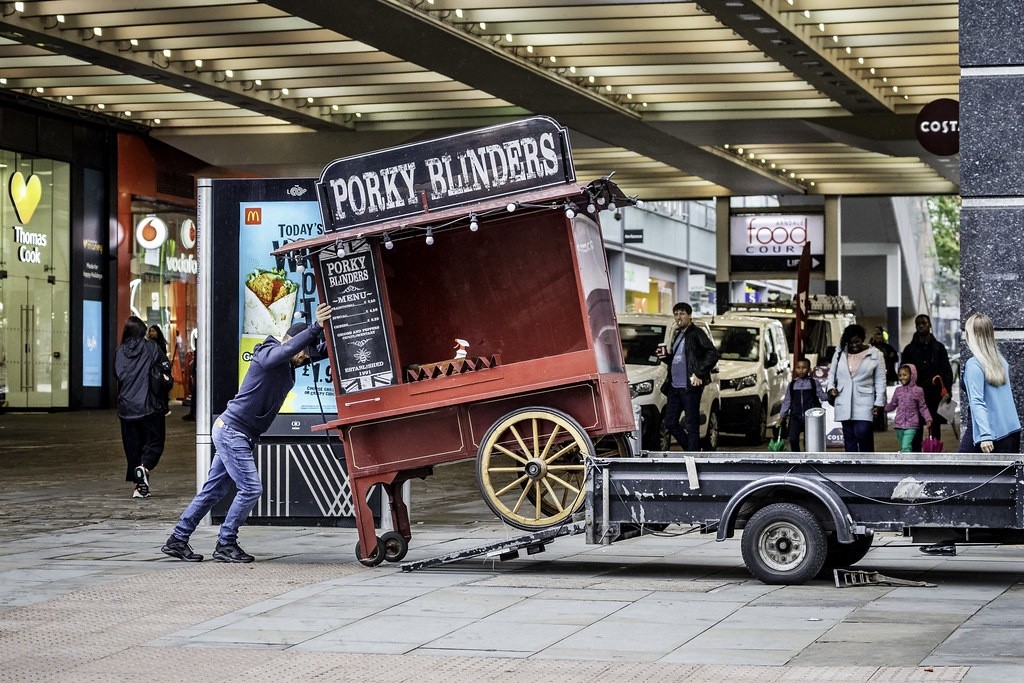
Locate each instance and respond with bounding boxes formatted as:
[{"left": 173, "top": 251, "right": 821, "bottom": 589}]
[
  {"left": 212, "top": 541, "right": 254, "bottom": 563},
  {"left": 133, "top": 485, "right": 151, "bottom": 497},
  {"left": 161, "top": 535, "right": 203, "bottom": 562},
  {"left": 134, "top": 464, "right": 149, "bottom": 495}
]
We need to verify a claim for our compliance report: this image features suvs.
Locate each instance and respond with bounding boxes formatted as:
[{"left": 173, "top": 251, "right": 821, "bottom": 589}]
[
  {"left": 705, "top": 314, "right": 792, "bottom": 447},
  {"left": 606, "top": 312, "right": 721, "bottom": 453},
  {"left": 716, "top": 309, "right": 861, "bottom": 377}
]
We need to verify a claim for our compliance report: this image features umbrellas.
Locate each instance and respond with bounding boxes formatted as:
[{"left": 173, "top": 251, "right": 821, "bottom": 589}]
[
  {"left": 768, "top": 424, "right": 787, "bottom": 451},
  {"left": 933, "top": 375, "right": 960, "bottom": 440},
  {"left": 921, "top": 423, "right": 943, "bottom": 453}
]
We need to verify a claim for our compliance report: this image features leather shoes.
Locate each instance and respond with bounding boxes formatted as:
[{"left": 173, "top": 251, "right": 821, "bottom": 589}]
[{"left": 919, "top": 543, "right": 956, "bottom": 556}]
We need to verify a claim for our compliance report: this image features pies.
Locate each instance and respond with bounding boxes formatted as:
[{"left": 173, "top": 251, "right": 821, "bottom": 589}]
[{"left": 243, "top": 267, "right": 302, "bottom": 335}]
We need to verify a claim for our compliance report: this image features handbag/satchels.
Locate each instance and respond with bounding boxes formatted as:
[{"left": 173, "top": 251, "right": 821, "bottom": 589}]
[
  {"left": 825, "top": 350, "right": 843, "bottom": 407},
  {"left": 149, "top": 346, "right": 174, "bottom": 414},
  {"left": 660, "top": 375, "right": 670, "bottom": 397}
]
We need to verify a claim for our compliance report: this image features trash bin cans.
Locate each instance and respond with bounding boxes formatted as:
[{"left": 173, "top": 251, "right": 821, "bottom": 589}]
[{"left": 874, "top": 388, "right": 888, "bottom": 431}]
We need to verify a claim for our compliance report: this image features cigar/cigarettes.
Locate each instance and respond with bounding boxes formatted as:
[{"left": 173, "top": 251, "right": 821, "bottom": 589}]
[{"left": 679, "top": 321, "right": 681, "bottom": 325}]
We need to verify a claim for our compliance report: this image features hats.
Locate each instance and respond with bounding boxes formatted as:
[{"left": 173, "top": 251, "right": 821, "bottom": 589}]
[{"left": 287, "top": 323, "right": 321, "bottom": 356}]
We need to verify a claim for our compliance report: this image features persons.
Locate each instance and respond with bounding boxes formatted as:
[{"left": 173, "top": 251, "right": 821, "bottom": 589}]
[
  {"left": 148, "top": 325, "right": 197, "bottom": 422},
  {"left": 883, "top": 364, "right": 932, "bottom": 452},
  {"left": 161, "top": 303, "right": 332, "bottom": 563},
  {"left": 116, "top": 316, "right": 170, "bottom": 498},
  {"left": 656, "top": 302, "right": 721, "bottom": 452},
  {"left": 779, "top": 359, "right": 839, "bottom": 452},
  {"left": 868, "top": 328, "right": 900, "bottom": 387},
  {"left": 958, "top": 312, "right": 1022, "bottom": 453},
  {"left": 827, "top": 324, "right": 887, "bottom": 453},
  {"left": 901, "top": 314, "right": 953, "bottom": 453}
]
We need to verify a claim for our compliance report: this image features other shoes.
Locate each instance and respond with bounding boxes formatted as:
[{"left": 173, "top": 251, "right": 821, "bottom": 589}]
[{"left": 181, "top": 414, "right": 196, "bottom": 421}]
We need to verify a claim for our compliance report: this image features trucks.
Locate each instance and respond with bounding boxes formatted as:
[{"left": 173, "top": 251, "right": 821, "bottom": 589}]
[{"left": 399, "top": 447, "right": 1024, "bottom": 587}]
